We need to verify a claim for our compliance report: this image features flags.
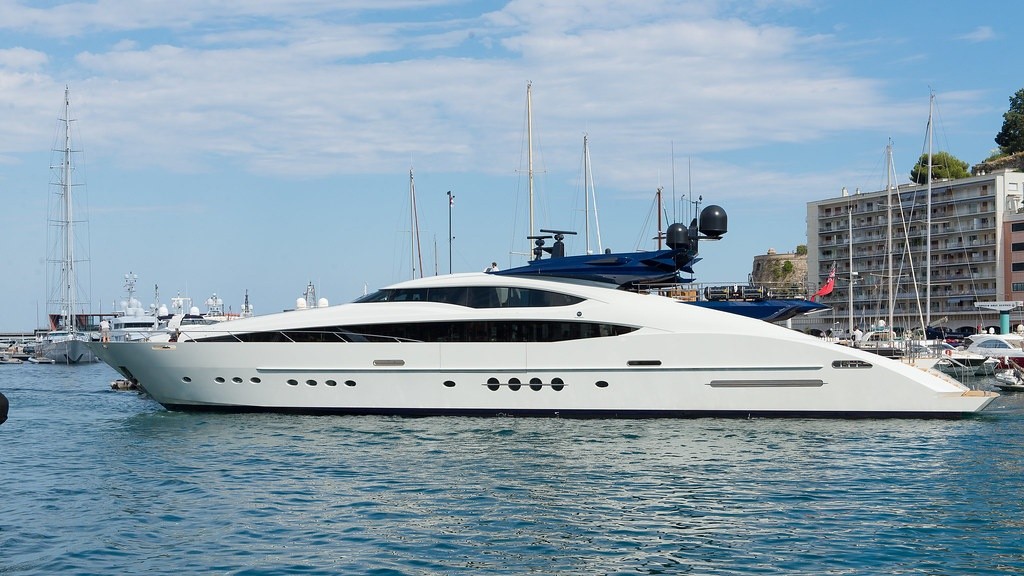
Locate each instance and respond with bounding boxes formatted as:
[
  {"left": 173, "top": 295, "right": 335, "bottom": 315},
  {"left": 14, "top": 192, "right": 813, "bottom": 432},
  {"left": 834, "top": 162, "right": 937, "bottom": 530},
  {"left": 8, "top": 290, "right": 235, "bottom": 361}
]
[
  {"left": 450, "top": 195, "right": 455, "bottom": 208},
  {"left": 810, "top": 260, "right": 836, "bottom": 302}
]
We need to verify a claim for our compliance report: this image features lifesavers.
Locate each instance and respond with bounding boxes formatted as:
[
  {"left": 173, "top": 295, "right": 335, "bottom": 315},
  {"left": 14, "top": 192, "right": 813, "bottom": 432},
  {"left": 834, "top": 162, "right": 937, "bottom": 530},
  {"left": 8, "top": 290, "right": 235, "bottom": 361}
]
[{"left": 945, "top": 349, "right": 952, "bottom": 356}]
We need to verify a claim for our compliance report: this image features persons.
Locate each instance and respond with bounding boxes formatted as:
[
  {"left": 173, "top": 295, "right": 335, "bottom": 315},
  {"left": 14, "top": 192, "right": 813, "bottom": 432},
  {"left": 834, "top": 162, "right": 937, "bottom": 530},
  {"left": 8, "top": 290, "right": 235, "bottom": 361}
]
[
  {"left": 100, "top": 319, "right": 111, "bottom": 342},
  {"left": 489, "top": 261, "right": 500, "bottom": 272},
  {"left": 853, "top": 326, "right": 863, "bottom": 340}
]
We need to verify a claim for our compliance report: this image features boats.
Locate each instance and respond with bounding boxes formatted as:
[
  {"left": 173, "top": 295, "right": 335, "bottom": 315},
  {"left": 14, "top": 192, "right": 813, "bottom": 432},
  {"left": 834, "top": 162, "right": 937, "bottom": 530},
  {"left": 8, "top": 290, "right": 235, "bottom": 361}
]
[
  {"left": 992, "top": 370, "right": 1024, "bottom": 392},
  {"left": 856, "top": 88, "right": 988, "bottom": 359},
  {"left": 882, "top": 149, "right": 1004, "bottom": 377},
  {"left": 87, "top": 75, "right": 999, "bottom": 418},
  {"left": 10, "top": 351, "right": 36, "bottom": 362},
  {"left": 832, "top": 207, "right": 865, "bottom": 346},
  {"left": 960, "top": 335, "right": 1024, "bottom": 371},
  {"left": 39, "top": 84, "right": 101, "bottom": 364}
]
